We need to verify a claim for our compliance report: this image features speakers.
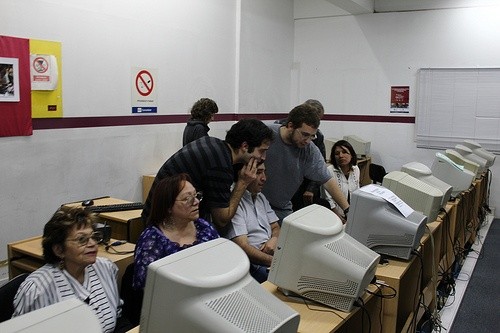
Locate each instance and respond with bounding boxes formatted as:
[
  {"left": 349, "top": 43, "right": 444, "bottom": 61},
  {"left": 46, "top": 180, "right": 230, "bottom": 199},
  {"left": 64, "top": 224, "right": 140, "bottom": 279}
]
[{"left": 94, "top": 223, "right": 112, "bottom": 244}]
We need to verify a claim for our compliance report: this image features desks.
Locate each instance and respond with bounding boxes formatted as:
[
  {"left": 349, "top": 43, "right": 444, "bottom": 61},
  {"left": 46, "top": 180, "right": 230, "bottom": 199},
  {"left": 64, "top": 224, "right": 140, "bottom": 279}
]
[
  {"left": 60, "top": 196, "right": 146, "bottom": 244},
  {"left": 7, "top": 235, "right": 136, "bottom": 291},
  {"left": 128, "top": 157, "right": 489, "bottom": 333}
]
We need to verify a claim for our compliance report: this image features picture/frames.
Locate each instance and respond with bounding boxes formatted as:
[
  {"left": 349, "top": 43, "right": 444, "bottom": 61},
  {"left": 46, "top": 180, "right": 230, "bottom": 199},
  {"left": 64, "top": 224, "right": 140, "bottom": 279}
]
[{"left": 0, "top": 56, "right": 21, "bottom": 102}]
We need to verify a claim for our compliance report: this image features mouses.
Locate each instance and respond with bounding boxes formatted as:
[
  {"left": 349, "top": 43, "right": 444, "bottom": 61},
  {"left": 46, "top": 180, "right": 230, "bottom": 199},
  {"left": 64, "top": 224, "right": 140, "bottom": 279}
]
[{"left": 82, "top": 200, "right": 93, "bottom": 206}]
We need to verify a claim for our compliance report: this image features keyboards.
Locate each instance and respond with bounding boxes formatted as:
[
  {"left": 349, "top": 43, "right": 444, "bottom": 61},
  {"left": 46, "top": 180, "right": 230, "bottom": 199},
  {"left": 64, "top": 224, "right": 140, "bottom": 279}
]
[{"left": 86, "top": 202, "right": 143, "bottom": 212}]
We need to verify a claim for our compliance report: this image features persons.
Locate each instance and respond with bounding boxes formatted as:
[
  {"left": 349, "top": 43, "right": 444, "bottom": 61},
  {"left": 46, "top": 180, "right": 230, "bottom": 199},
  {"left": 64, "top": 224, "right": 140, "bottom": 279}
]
[
  {"left": 182, "top": 98, "right": 219, "bottom": 147},
  {"left": 218, "top": 158, "right": 280, "bottom": 284},
  {"left": 141, "top": 118, "right": 275, "bottom": 228},
  {"left": 264, "top": 103, "right": 350, "bottom": 229},
  {"left": 132, "top": 172, "right": 221, "bottom": 325},
  {"left": 274, "top": 99, "right": 326, "bottom": 212},
  {"left": 11, "top": 205, "right": 124, "bottom": 333},
  {"left": 320, "top": 140, "right": 360, "bottom": 226}
]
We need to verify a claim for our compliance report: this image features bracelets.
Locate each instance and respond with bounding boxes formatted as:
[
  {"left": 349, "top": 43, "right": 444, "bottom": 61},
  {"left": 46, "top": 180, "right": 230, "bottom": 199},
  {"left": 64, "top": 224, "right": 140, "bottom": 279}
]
[{"left": 343, "top": 206, "right": 349, "bottom": 215}]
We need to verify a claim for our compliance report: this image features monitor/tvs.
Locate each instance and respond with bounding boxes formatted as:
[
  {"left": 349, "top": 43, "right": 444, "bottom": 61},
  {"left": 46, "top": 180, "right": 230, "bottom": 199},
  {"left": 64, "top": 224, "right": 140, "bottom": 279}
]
[
  {"left": 0, "top": 298, "right": 104, "bottom": 333},
  {"left": 345, "top": 184, "right": 427, "bottom": 260},
  {"left": 401, "top": 140, "right": 496, "bottom": 213},
  {"left": 325, "top": 135, "right": 371, "bottom": 162},
  {"left": 139, "top": 238, "right": 300, "bottom": 333},
  {"left": 267, "top": 204, "right": 381, "bottom": 313},
  {"left": 382, "top": 170, "right": 443, "bottom": 223}
]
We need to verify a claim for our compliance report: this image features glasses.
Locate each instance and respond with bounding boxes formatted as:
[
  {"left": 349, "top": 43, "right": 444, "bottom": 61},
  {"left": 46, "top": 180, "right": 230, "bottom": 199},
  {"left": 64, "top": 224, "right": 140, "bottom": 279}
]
[
  {"left": 296, "top": 130, "right": 317, "bottom": 140},
  {"left": 176, "top": 191, "right": 203, "bottom": 203},
  {"left": 65, "top": 231, "right": 103, "bottom": 246}
]
